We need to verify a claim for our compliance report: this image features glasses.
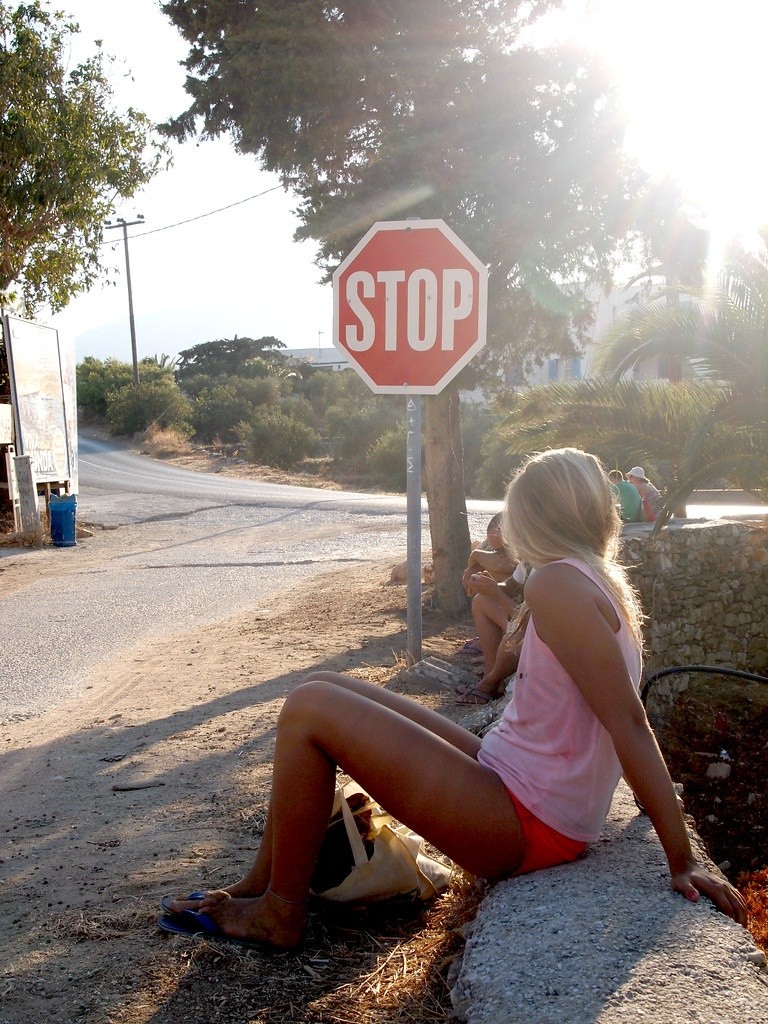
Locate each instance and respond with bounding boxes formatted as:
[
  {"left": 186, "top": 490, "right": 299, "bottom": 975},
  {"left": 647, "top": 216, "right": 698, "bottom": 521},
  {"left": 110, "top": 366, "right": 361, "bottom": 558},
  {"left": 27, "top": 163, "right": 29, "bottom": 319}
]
[{"left": 492, "top": 548, "right": 505, "bottom": 558}]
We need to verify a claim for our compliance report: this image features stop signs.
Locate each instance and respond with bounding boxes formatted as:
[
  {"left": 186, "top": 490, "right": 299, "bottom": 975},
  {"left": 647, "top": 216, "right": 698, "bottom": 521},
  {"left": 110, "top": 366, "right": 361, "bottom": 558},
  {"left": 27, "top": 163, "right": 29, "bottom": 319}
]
[{"left": 333, "top": 218, "right": 488, "bottom": 394}]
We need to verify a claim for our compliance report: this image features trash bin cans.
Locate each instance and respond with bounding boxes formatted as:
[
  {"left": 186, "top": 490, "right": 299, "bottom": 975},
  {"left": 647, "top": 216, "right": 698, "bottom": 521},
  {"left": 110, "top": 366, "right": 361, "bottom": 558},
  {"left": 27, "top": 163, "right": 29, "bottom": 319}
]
[{"left": 49, "top": 493, "right": 78, "bottom": 547}]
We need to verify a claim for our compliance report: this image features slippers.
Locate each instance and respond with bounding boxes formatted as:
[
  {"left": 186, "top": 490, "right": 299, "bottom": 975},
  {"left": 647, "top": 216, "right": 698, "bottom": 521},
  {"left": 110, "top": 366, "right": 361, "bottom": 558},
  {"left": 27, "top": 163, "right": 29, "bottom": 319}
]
[
  {"left": 458, "top": 644, "right": 484, "bottom": 656},
  {"left": 455, "top": 681, "right": 506, "bottom": 707},
  {"left": 155, "top": 892, "right": 304, "bottom": 955}
]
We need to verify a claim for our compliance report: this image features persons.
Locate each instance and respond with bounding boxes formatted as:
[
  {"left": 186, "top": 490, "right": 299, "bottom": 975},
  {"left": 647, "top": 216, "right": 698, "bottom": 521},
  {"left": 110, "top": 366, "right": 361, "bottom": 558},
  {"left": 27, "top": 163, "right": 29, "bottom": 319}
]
[
  {"left": 609, "top": 466, "right": 664, "bottom": 522},
  {"left": 169, "top": 447, "right": 748, "bottom": 945},
  {"left": 455, "top": 510, "right": 530, "bottom": 705}
]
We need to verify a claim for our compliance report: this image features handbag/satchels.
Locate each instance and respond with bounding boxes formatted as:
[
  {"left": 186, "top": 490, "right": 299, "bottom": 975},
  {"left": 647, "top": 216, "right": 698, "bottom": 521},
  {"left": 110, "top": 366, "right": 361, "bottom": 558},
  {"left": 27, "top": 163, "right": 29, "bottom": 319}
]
[{"left": 306, "top": 779, "right": 458, "bottom": 906}]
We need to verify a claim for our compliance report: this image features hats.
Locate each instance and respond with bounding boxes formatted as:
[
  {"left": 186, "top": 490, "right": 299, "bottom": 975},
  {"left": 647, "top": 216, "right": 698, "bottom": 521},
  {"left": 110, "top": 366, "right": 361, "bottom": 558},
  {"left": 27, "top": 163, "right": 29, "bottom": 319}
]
[{"left": 625, "top": 467, "right": 649, "bottom": 481}]
